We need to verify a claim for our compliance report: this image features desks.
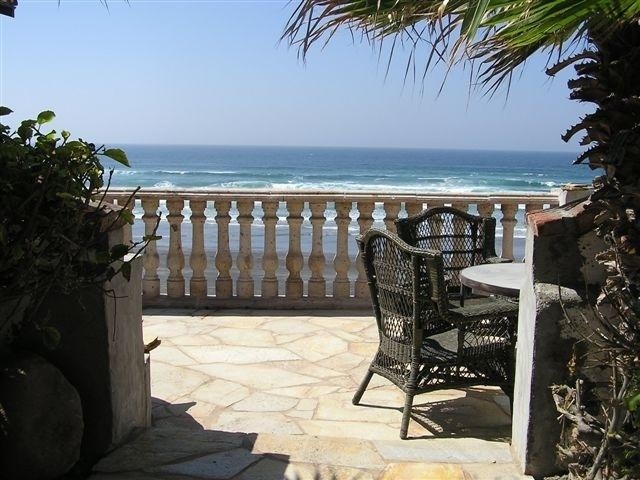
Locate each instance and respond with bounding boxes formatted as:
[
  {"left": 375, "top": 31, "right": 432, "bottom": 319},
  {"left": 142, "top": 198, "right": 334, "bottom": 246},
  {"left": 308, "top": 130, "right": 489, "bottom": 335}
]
[{"left": 455, "top": 262, "right": 525, "bottom": 379}]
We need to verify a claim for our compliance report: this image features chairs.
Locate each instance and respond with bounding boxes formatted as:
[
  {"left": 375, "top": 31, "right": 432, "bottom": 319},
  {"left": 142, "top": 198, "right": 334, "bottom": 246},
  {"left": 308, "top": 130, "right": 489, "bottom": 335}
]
[
  {"left": 351, "top": 227, "right": 520, "bottom": 440},
  {"left": 395, "top": 206, "right": 520, "bottom": 339}
]
